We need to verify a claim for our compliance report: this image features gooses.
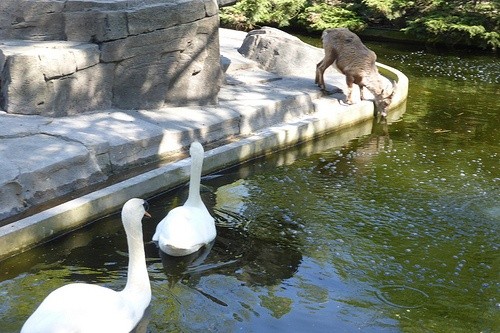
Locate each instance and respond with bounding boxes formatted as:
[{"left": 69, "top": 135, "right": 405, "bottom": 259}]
[
  {"left": 19, "top": 198, "right": 153, "bottom": 333},
  {"left": 151, "top": 140, "right": 217, "bottom": 257}
]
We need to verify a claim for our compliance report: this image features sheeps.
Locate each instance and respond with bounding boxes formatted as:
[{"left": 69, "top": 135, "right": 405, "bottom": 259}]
[{"left": 314, "top": 27, "right": 394, "bottom": 119}]
